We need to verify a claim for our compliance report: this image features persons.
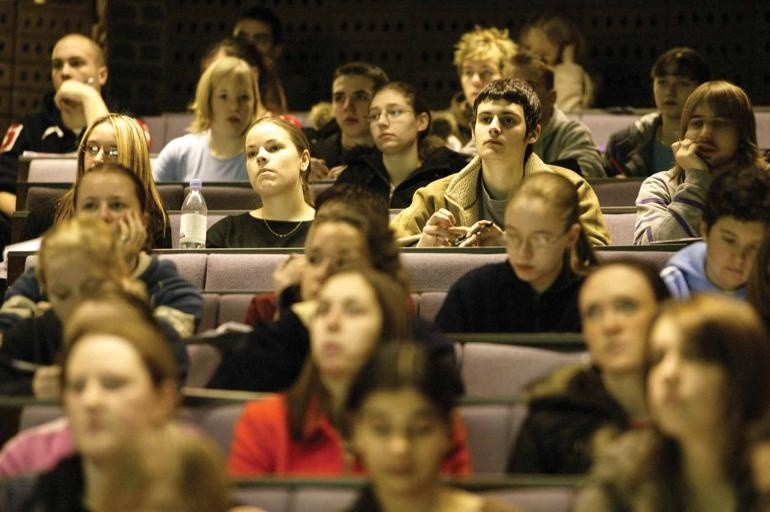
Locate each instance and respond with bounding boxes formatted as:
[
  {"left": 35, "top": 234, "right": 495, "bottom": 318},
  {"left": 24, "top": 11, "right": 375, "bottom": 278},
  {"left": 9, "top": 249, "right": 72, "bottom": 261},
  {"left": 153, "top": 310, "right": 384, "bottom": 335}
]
[{"left": 0, "top": 15, "right": 770, "bottom": 512}]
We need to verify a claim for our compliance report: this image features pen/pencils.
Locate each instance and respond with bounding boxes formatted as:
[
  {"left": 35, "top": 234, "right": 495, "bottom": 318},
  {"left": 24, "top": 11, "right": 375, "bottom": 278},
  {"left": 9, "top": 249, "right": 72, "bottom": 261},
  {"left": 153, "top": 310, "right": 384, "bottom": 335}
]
[{"left": 454, "top": 223, "right": 493, "bottom": 245}]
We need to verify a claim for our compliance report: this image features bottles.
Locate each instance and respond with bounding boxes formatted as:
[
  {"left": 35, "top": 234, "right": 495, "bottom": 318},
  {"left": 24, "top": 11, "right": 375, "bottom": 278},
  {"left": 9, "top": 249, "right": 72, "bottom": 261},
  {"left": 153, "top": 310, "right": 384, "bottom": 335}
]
[{"left": 178, "top": 180, "right": 208, "bottom": 249}]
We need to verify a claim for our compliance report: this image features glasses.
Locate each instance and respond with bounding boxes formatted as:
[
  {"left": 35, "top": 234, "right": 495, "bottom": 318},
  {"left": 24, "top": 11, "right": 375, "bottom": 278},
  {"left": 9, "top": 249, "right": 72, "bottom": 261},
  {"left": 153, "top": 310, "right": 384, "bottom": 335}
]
[
  {"left": 366, "top": 108, "right": 413, "bottom": 122},
  {"left": 80, "top": 145, "right": 118, "bottom": 160},
  {"left": 501, "top": 229, "right": 569, "bottom": 254}
]
